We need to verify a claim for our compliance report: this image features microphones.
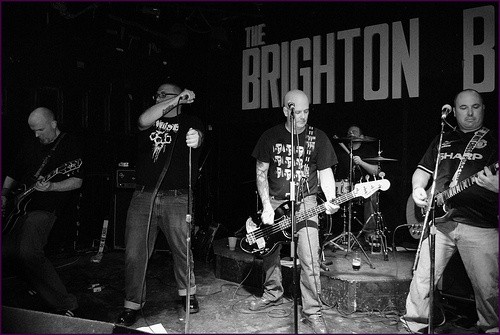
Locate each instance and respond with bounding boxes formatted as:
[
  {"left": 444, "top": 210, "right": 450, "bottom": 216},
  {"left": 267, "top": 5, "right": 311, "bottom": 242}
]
[
  {"left": 441, "top": 104, "right": 452, "bottom": 120},
  {"left": 287, "top": 102, "right": 295, "bottom": 117},
  {"left": 179, "top": 95, "right": 188, "bottom": 101}
]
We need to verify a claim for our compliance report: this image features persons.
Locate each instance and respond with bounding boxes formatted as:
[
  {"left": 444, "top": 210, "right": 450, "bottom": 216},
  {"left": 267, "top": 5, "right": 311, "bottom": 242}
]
[
  {"left": 0, "top": 106, "right": 85, "bottom": 320},
  {"left": 248, "top": 88, "right": 380, "bottom": 333},
  {"left": 400, "top": 89, "right": 500, "bottom": 333},
  {"left": 117, "top": 80, "right": 212, "bottom": 329}
]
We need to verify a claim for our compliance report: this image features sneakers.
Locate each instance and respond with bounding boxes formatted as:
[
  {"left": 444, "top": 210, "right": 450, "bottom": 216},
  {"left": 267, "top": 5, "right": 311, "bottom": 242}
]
[
  {"left": 248, "top": 296, "right": 284, "bottom": 311},
  {"left": 117, "top": 308, "right": 141, "bottom": 325},
  {"left": 301, "top": 313, "right": 327, "bottom": 335},
  {"left": 181, "top": 294, "right": 199, "bottom": 314}
]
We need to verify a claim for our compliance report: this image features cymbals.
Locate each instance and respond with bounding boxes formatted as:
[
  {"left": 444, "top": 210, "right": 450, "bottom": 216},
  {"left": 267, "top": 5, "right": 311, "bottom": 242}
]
[
  {"left": 362, "top": 157, "right": 398, "bottom": 162},
  {"left": 332, "top": 136, "right": 377, "bottom": 142}
]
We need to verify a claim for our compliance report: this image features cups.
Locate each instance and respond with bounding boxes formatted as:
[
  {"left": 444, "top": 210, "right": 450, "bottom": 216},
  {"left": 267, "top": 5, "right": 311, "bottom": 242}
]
[
  {"left": 351, "top": 251, "right": 361, "bottom": 271},
  {"left": 228, "top": 237, "right": 237, "bottom": 250}
]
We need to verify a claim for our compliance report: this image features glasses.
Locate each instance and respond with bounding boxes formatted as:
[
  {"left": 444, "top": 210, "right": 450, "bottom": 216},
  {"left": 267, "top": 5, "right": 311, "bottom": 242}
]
[{"left": 152, "top": 92, "right": 178, "bottom": 101}]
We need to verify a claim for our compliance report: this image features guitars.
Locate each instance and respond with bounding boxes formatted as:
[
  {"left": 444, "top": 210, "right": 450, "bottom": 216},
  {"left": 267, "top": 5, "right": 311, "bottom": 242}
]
[
  {"left": 0, "top": 157, "right": 83, "bottom": 234},
  {"left": 245, "top": 171, "right": 391, "bottom": 257},
  {"left": 406, "top": 160, "right": 500, "bottom": 240}
]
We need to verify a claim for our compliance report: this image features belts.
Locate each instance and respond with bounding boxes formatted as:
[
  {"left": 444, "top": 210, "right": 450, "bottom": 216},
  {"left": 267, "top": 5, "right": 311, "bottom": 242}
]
[{"left": 134, "top": 184, "right": 189, "bottom": 196}]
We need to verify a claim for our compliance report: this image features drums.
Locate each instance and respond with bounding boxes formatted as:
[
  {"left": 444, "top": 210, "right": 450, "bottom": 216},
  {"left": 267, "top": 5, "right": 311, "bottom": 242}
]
[
  {"left": 336, "top": 181, "right": 364, "bottom": 205},
  {"left": 317, "top": 194, "right": 331, "bottom": 244}
]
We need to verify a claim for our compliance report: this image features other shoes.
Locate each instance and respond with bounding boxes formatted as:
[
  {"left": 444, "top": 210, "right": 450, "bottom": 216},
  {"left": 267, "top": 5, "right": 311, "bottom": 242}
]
[
  {"left": 366, "top": 235, "right": 380, "bottom": 248},
  {"left": 399, "top": 327, "right": 418, "bottom": 335},
  {"left": 480, "top": 326, "right": 500, "bottom": 335},
  {"left": 23, "top": 287, "right": 40, "bottom": 299},
  {"left": 64, "top": 304, "right": 80, "bottom": 317}
]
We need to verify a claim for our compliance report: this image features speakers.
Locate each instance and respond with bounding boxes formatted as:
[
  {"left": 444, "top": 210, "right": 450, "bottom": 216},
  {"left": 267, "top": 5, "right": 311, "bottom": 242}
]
[
  {"left": 115, "top": 188, "right": 135, "bottom": 249},
  {"left": 1, "top": 305, "right": 150, "bottom": 334}
]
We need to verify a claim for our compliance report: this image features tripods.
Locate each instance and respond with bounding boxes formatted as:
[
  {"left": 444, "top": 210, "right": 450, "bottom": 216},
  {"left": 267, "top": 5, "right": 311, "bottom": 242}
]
[{"left": 321, "top": 141, "right": 391, "bottom": 269}]
